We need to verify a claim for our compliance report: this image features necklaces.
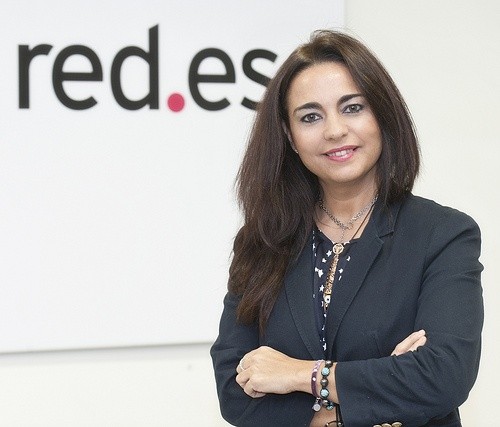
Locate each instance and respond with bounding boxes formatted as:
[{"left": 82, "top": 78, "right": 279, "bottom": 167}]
[{"left": 317, "top": 189, "right": 380, "bottom": 230}]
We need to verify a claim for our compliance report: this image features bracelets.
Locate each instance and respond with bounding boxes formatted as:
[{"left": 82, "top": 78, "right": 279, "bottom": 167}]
[{"left": 310, "top": 359, "right": 344, "bottom": 427}]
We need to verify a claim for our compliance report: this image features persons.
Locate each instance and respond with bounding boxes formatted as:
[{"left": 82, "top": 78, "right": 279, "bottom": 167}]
[{"left": 210, "top": 31, "right": 484, "bottom": 427}]
